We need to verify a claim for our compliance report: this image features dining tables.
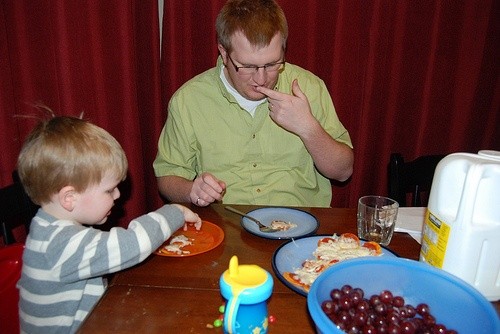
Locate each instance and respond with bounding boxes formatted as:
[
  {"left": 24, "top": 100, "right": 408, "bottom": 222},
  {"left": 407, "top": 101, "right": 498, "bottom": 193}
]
[{"left": 74, "top": 204, "right": 421, "bottom": 334}]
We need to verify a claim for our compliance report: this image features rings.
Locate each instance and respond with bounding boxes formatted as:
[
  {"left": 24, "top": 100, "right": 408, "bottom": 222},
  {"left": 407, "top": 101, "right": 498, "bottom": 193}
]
[{"left": 196, "top": 197, "right": 200, "bottom": 205}]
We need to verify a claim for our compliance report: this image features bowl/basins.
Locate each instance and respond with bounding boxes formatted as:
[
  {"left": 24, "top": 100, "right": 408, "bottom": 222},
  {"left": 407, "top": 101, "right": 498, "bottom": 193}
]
[{"left": 306, "top": 254, "right": 500, "bottom": 334}]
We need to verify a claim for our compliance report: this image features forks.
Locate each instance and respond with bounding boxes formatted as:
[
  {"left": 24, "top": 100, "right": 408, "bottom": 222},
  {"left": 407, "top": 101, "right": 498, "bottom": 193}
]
[{"left": 224, "top": 205, "right": 279, "bottom": 233}]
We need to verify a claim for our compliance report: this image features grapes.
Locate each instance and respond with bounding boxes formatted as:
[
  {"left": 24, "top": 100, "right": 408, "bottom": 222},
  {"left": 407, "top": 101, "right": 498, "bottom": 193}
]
[{"left": 322, "top": 285, "right": 459, "bottom": 334}]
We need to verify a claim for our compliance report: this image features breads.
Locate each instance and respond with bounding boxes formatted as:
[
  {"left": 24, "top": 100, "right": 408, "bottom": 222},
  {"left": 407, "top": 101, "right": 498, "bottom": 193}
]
[
  {"left": 283, "top": 272, "right": 312, "bottom": 292},
  {"left": 319, "top": 233, "right": 381, "bottom": 254}
]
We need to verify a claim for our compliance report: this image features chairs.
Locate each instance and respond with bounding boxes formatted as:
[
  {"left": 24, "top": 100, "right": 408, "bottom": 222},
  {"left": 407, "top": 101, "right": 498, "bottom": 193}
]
[
  {"left": 0, "top": 171, "right": 40, "bottom": 246},
  {"left": 386, "top": 152, "right": 445, "bottom": 207}
]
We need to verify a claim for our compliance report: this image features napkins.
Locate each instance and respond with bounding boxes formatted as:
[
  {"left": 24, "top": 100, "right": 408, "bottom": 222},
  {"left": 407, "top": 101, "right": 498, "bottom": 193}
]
[{"left": 393, "top": 207, "right": 427, "bottom": 244}]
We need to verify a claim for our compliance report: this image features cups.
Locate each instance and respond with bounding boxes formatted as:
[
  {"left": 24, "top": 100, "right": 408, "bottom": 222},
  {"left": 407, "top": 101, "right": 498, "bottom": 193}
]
[{"left": 357, "top": 196, "right": 399, "bottom": 247}]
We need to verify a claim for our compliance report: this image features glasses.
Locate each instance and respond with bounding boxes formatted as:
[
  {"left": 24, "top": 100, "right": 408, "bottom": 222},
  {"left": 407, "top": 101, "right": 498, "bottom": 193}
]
[{"left": 226, "top": 47, "right": 285, "bottom": 75}]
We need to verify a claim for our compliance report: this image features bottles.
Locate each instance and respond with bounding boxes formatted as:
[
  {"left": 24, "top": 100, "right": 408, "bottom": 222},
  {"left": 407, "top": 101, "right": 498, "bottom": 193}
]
[
  {"left": 218, "top": 255, "right": 275, "bottom": 334},
  {"left": 419, "top": 149, "right": 500, "bottom": 304}
]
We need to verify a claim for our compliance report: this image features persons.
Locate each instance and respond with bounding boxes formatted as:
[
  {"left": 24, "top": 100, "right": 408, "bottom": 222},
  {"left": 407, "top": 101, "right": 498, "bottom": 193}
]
[
  {"left": 13, "top": 100, "right": 202, "bottom": 334},
  {"left": 153, "top": 0, "right": 353, "bottom": 206}
]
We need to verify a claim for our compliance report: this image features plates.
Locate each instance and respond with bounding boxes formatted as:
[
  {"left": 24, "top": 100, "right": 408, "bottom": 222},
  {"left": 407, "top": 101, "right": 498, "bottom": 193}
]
[
  {"left": 240, "top": 207, "right": 320, "bottom": 240},
  {"left": 272, "top": 233, "right": 400, "bottom": 296},
  {"left": 152, "top": 219, "right": 225, "bottom": 256}
]
